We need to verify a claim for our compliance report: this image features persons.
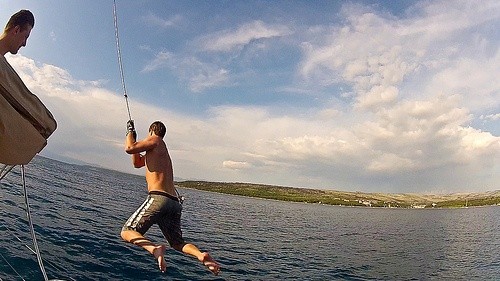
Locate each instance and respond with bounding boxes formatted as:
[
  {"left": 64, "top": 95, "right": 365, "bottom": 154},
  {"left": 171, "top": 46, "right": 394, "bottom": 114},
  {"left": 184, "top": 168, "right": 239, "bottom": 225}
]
[
  {"left": 121, "top": 120, "right": 221, "bottom": 277},
  {"left": 0, "top": 7, "right": 36, "bottom": 58}
]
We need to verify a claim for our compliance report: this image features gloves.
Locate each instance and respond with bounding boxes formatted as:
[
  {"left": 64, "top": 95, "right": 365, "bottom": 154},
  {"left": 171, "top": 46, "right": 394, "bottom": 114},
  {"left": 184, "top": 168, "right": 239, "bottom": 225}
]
[{"left": 126, "top": 120, "right": 134, "bottom": 133}]
